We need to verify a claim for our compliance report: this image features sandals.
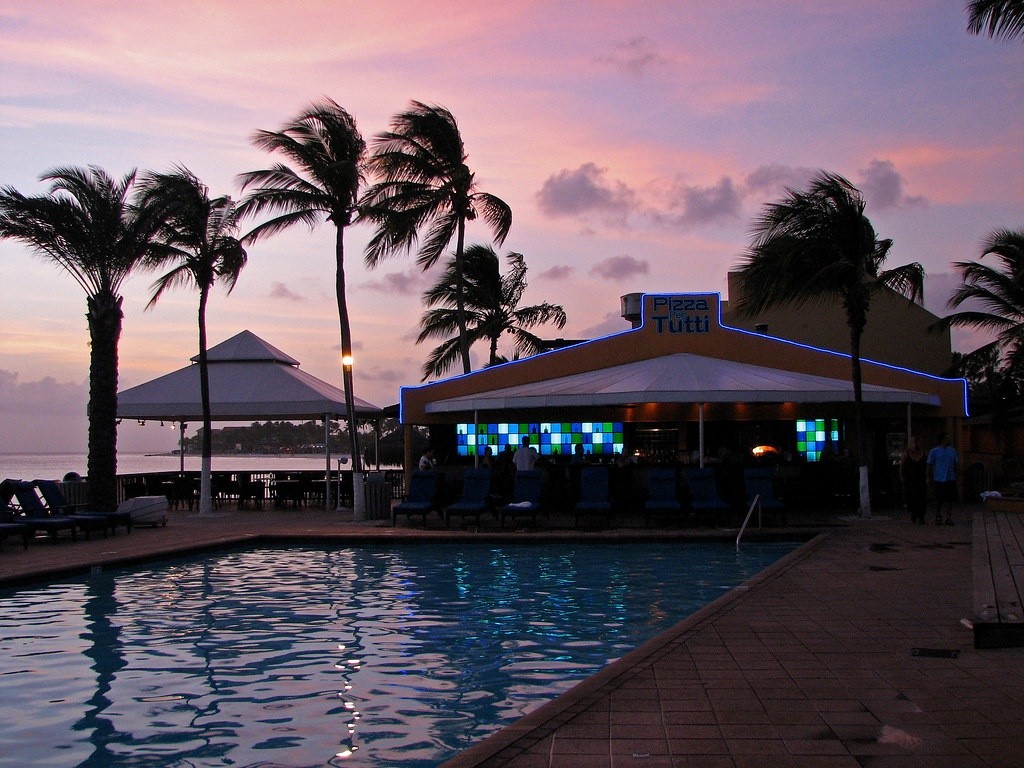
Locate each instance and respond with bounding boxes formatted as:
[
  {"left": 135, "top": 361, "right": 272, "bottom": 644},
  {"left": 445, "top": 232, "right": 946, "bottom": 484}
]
[
  {"left": 936, "top": 513, "right": 942, "bottom": 525},
  {"left": 945, "top": 518, "right": 954, "bottom": 526}
]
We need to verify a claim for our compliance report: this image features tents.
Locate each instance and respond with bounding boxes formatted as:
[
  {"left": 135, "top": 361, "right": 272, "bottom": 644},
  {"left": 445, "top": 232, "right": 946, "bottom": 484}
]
[{"left": 85, "top": 330, "right": 381, "bottom": 510}]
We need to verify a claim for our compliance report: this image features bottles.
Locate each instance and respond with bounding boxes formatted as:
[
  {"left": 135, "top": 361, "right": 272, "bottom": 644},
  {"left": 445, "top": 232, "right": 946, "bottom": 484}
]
[
  {"left": 650, "top": 448, "right": 668, "bottom": 458},
  {"left": 457, "top": 458, "right": 465, "bottom": 485},
  {"left": 446, "top": 461, "right": 456, "bottom": 490}
]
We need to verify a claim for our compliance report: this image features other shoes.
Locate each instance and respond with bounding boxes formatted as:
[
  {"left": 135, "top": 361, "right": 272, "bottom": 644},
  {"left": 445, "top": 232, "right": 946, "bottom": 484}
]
[{"left": 911, "top": 513, "right": 925, "bottom": 524}]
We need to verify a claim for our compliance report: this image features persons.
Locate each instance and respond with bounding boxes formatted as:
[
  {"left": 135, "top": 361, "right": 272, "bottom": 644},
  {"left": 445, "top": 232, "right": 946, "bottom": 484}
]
[
  {"left": 898, "top": 435, "right": 929, "bottom": 525},
  {"left": 923, "top": 432, "right": 962, "bottom": 526},
  {"left": 417, "top": 433, "right": 588, "bottom": 479}
]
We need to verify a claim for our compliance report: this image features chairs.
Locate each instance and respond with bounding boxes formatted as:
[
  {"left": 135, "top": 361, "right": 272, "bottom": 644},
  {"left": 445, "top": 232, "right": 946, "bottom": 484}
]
[
  {"left": 144, "top": 473, "right": 263, "bottom": 520},
  {"left": 392, "top": 469, "right": 615, "bottom": 530},
  {"left": 646, "top": 469, "right": 787, "bottom": 528},
  {"left": 269, "top": 471, "right": 354, "bottom": 511},
  {"left": 0, "top": 479, "right": 132, "bottom": 553}
]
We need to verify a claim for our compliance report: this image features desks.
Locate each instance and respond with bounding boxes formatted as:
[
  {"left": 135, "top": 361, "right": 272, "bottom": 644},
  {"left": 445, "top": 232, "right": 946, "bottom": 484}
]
[
  {"left": 276, "top": 480, "right": 300, "bottom": 509},
  {"left": 312, "top": 480, "right": 339, "bottom": 504}
]
